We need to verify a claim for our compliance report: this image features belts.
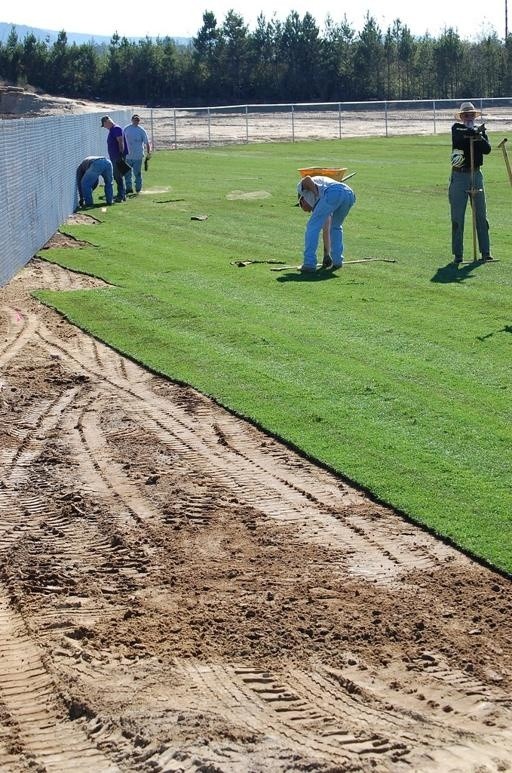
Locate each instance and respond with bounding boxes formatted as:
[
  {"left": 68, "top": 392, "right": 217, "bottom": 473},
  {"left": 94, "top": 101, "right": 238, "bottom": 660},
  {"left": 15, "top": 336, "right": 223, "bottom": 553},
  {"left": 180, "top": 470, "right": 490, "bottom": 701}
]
[{"left": 453, "top": 167, "right": 479, "bottom": 172}]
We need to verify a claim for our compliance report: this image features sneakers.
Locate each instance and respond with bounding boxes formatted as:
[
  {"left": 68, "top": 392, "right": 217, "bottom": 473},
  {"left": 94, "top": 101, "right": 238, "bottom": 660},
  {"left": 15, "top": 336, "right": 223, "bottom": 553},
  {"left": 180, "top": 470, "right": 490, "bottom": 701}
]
[
  {"left": 297, "top": 264, "right": 316, "bottom": 273},
  {"left": 455, "top": 255, "right": 463, "bottom": 264},
  {"left": 327, "top": 264, "right": 341, "bottom": 273},
  {"left": 482, "top": 255, "right": 500, "bottom": 261},
  {"left": 86, "top": 187, "right": 140, "bottom": 207}
]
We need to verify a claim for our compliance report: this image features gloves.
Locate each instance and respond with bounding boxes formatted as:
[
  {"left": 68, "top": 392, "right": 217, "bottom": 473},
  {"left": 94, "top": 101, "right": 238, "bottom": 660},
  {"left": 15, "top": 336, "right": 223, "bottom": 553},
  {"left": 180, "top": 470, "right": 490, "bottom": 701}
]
[
  {"left": 323, "top": 254, "right": 332, "bottom": 268},
  {"left": 146, "top": 151, "right": 152, "bottom": 159}
]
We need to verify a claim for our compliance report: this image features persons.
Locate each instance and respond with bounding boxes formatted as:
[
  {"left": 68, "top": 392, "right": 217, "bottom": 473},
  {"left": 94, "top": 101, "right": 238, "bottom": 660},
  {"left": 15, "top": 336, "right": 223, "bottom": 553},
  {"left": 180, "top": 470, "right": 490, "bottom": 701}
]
[
  {"left": 75, "top": 155, "right": 114, "bottom": 207},
  {"left": 295, "top": 173, "right": 357, "bottom": 273},
  {"left": 447, "top": 101, "right": 494, "bottom": 263},
  {"left": 122, "top": 113, "right": 152, "bottom": 194},
  {"left": 99, "top": 114, "right": 132, "bottom": 203}
]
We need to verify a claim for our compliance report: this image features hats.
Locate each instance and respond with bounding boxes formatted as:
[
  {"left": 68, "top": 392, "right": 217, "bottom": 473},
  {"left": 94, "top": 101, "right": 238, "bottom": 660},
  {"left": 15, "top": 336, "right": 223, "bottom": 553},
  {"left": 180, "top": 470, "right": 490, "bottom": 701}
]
[
  {"left": 453, "top": 102, "right": 481, "bottom": 122},
  {"left": 100, "top": 116, "right": 109, "bottom": 127},
  {"left": 132, "top": 114, "right": 140, "bottom": 120}
]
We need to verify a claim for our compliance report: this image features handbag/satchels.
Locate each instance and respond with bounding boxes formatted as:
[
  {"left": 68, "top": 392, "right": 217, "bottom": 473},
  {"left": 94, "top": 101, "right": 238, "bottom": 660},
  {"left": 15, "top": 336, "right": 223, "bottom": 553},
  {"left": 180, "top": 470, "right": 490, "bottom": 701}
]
[{"left": 117, "top": 151, "right": 131, "bottom": 174}]
